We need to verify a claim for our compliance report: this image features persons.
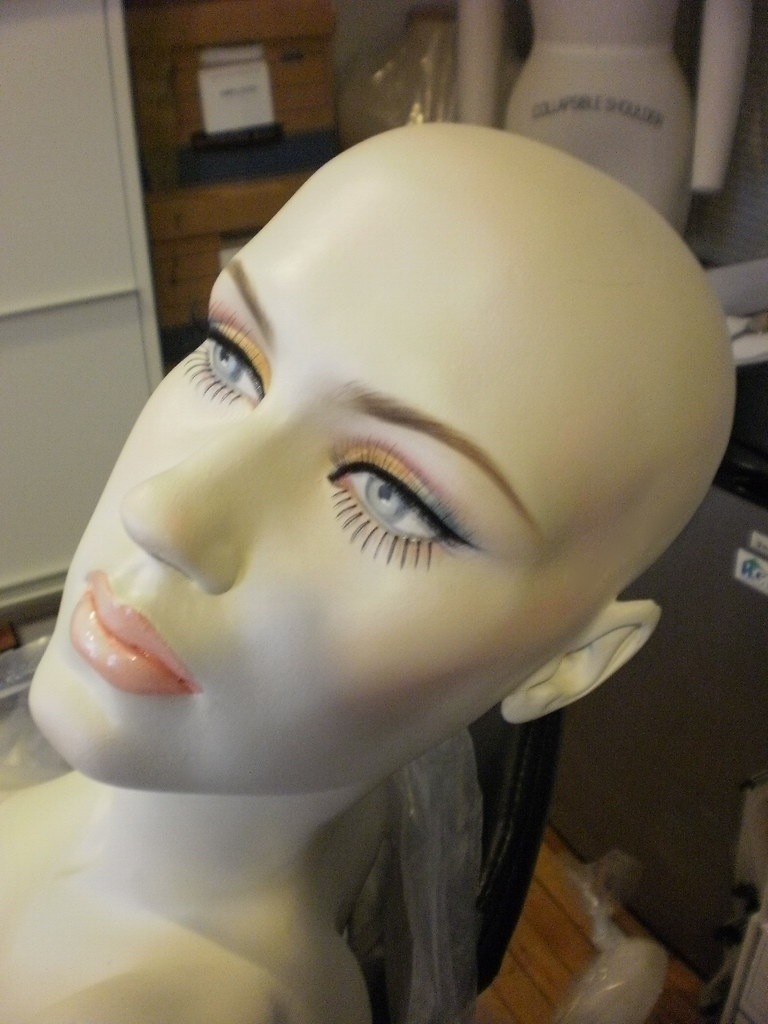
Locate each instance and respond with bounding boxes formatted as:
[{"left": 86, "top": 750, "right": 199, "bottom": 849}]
[
  {"left": 1, "top": 117, "right": 738, "bottom": 1024},
  {"left": 454, "top": 1, "right": 754, "bottom": 241}
]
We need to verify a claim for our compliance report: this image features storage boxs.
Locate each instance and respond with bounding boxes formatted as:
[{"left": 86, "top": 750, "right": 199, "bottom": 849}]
[
  {"left": 138, "top": 132, "right": 345, "bottom": 333},
  {"left": 123, "top": 0, "right": 338, "bottom": 192}
]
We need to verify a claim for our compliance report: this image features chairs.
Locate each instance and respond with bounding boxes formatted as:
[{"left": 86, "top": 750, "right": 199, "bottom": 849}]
[{"left": 301, "top": 700, "right": 564, "bottom": 1024}]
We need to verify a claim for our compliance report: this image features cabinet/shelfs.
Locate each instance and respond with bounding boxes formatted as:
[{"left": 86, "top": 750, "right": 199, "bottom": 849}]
[{"left": 1, "top": 0, "right": 167, "bottom": 627}]
